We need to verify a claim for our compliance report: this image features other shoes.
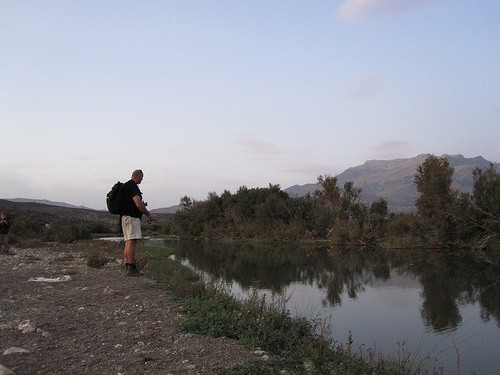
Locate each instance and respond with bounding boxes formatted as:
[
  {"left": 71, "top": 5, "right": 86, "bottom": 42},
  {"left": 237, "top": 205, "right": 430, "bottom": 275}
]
[{"left": 125, "top": 265, "right": 144, "bottom": 277}]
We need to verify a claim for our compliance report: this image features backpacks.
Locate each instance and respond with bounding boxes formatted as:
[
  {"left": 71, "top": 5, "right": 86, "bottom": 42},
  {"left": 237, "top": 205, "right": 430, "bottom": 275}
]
[{"left": 105, "top": 181, "right": 133, "bottom": 215}]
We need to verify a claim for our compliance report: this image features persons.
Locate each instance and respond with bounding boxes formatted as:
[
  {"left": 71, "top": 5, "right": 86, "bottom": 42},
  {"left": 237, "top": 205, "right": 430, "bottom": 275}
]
[{"left": 122, "top": 170, "right": 155, "bottom": 277}]
